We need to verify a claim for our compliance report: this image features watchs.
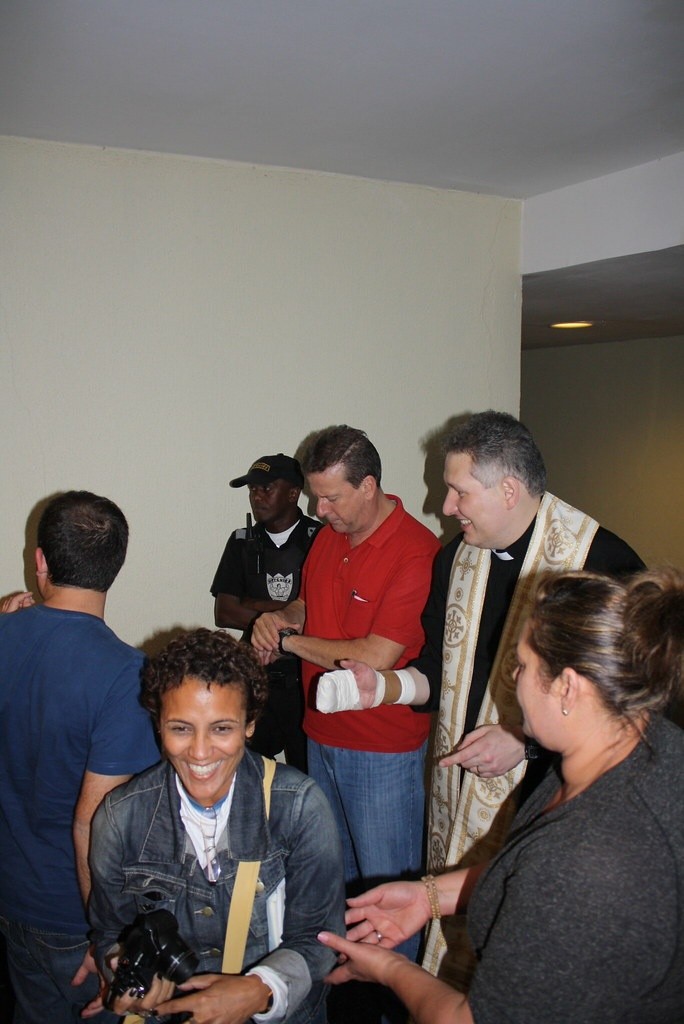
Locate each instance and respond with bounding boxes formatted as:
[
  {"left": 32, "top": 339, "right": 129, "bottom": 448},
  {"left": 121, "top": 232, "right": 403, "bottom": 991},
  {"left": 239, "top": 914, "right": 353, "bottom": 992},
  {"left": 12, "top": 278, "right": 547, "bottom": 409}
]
[
  {"left": 278, "top": 628, "right": 298, "bottom": 656},
  {"left": 526, "top": 736, "right": 540, "bottom": 760}
]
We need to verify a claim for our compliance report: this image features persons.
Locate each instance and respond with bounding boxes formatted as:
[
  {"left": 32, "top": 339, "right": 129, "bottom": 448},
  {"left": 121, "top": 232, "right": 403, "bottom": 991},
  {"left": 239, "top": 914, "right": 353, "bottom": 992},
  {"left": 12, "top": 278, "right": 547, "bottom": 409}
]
[
  {"left": 212, "top": 453, "right": 323, "bottom": 777},
  {"left": 0, "top": 492, "right": 164, "bottom": 1024},
  {"left": 316, "top": 412, "right": 648, "bottom": 1024},
  {"left": 251, "top": 424, "right": 442, "bottom": 1024},
  {"left": 318, "top": 569, "right": 684, "bottom": 1024},
  {"left": 87, "top": 629, "right": 347, "bottom": 1024}
]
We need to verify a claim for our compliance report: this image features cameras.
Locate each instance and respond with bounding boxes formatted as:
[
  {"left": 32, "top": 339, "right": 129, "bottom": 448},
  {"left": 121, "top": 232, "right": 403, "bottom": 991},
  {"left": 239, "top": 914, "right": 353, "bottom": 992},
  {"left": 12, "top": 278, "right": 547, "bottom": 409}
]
[{"left": 107, "top": 908, "right": 198, "bottom": 999}]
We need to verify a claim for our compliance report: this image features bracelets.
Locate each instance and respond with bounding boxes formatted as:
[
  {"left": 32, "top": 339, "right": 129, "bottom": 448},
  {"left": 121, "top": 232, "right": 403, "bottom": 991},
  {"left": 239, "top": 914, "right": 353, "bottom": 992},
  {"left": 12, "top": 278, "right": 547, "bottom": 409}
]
[{"left": 421, "top": 874, "right": 441, "bottom": 920}]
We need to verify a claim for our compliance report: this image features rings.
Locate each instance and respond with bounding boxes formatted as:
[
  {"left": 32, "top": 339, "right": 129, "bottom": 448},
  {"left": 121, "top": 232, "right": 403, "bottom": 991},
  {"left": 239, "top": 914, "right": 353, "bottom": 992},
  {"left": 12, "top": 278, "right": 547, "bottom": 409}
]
[
  {"left": 477, "top": 766, "right": 480, "bottom": 774},
  {"left": 374, "top": 930, "right": 382, "bottom": 941}
]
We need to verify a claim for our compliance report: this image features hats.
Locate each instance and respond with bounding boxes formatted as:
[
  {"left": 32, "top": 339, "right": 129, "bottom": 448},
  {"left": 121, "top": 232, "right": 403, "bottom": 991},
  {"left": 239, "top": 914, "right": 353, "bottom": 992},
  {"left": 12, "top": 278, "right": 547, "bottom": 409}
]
[{"left": 229, "top": 453, "right": 305, "bottom": 488}]
